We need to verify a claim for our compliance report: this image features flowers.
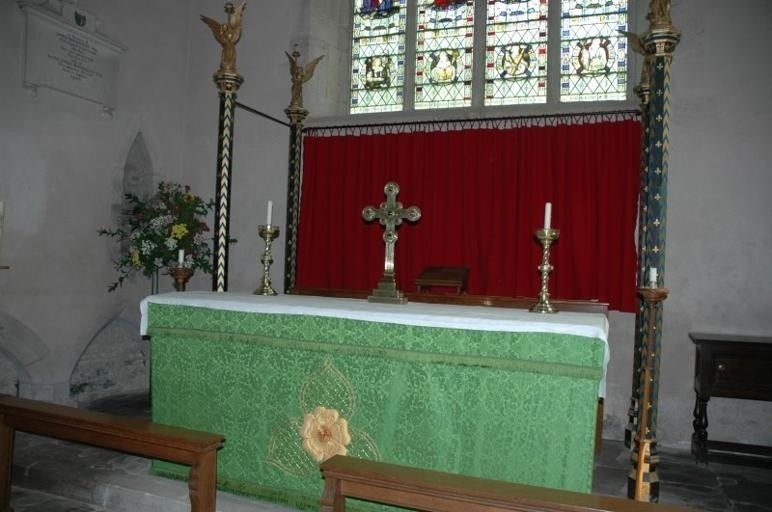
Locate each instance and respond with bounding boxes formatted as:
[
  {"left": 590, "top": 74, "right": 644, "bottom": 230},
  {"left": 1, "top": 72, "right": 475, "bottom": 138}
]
[{"left": 96, "top": 179, "right": 217, "bottom": 292}]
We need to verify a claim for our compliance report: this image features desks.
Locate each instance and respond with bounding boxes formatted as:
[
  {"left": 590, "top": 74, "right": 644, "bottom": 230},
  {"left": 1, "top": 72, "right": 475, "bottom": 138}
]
[
  {"left": 146, "top": 291, "right": 610, "bottom": 512},
  {"left": 689, "top": 331, "right": 772, "bottom": 468}
]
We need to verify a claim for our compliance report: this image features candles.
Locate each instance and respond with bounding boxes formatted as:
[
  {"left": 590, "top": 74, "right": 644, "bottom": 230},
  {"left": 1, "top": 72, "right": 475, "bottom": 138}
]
[
  {"left": 266, "top": 197, "right": 273, "bottom": 227},
  {"left": 648, "top": 266, "right": 658, "bottom": 289},
  {"left": 178, "top": 246, "right": 186, "bottom": 268},
  {"left": 544, "top": 200, "right": 552, "bottom": 230}
]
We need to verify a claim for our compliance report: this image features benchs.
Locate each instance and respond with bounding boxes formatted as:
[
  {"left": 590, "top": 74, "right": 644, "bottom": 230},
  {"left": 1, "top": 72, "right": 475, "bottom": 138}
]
[
  {"left": 319, "top": 455, "right": 708, "bottom": 512},
  {"left": 0, "top": 393, "right": 225, "bottom": 511}
]
